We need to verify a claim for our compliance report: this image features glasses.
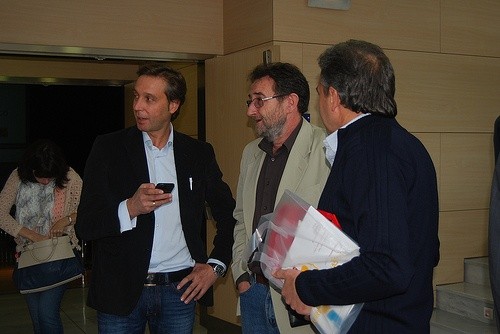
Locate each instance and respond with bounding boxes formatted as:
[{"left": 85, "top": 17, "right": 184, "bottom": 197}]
[{"left": 247, "top": 92, "right": 293, "bottom": 109}]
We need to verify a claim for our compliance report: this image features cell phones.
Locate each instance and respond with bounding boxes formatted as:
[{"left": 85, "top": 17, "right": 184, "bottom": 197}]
[{"left": 154, "top": 183, "right": 174, "bottom": 194}]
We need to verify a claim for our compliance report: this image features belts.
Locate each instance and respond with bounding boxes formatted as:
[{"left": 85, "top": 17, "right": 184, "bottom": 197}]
[
  {"left": 144, "top": 266, "right": 194, "bottom": 285},
  {"left": 249, "top": 271, "right": 270, "bottom": 283}
]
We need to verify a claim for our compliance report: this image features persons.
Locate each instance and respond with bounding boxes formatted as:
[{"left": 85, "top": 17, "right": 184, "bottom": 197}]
[
  {"left": 0, "top": 136, "right": 83, "bottom": 334},
  {"left": 75, "top": 62, "right": 237, "bottom": 334},
  {"left": 271, "top": 38, "right": 440, "bottom": 334},
  {"left": 230, "top": 61, "right": 336, "bottom": 334}
]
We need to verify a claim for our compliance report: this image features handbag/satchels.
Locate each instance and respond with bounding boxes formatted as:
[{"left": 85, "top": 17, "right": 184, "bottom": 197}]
[
  {"left": 243, "top": 189, "right": 369, "bottom": 334},
  {"left": 18, "top": 231, "right": 82, "bottom": 295}
]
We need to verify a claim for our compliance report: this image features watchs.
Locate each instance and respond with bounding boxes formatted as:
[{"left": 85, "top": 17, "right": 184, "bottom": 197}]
[
  {"left": 66, "top": 216, "right": 72, "bottom": 226},
  {"left": 208, "top": 262, "right": 225, "bottom": 277}
]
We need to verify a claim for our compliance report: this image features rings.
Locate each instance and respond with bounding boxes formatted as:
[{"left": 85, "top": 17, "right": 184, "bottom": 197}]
[{"left": 153, "top": 201, "right": 157, "bottom": 207}]
